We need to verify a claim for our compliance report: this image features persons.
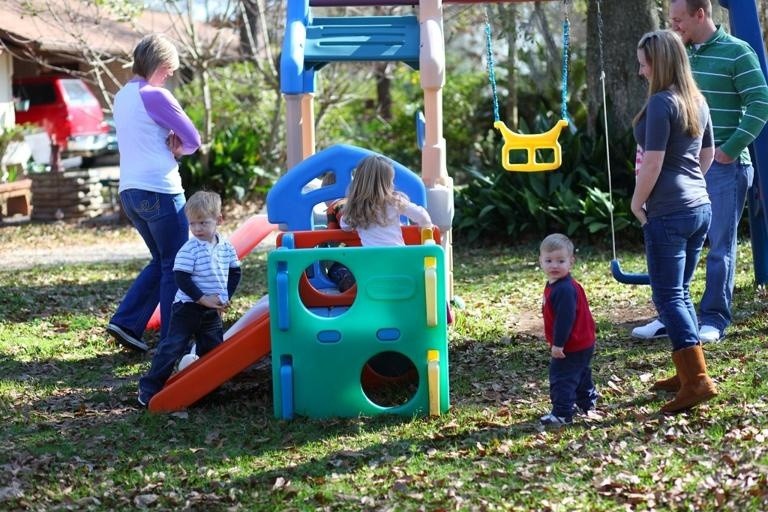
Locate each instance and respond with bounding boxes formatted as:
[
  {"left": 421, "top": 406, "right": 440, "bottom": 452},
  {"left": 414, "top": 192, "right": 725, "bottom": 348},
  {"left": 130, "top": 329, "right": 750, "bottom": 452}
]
[
  {"left": 632, "top": 0, "right": 768, "bottom": 343},
  {"left": 138, "top": 191, "right": 241, "bottom": 406},
  {"left": 630, "top": 29, "right": 718, "bottom": 413},
  {"left": 318, "top": 154, "right": 432, "bottom": 294},
  {"left": 539, "top": 233, "right": 596, "bottom": 424},
  {"left": 104, "top": 34, "right": 200, "bottom": 360}
]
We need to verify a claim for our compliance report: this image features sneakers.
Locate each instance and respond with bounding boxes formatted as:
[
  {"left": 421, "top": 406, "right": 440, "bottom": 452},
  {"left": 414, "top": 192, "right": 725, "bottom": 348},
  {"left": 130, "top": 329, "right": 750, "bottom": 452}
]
[
  {"left": 106, "top": 322, "right": 148, "bottom": 352},
  {"left": 541, "top": 404, "right": 596, "bottom": 427},
  {"left": 138, "top": 376, "right": 158, "bottom": 406},
  {"left": 631, "top": 320, "right": 669, "bottom": 340},
  {"left": 698, "top": 324, "right": 724, "bottom": 343}
]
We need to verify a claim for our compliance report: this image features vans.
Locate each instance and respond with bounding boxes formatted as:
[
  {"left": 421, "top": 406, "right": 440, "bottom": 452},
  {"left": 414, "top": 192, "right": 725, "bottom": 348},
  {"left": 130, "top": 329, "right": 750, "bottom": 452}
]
[{"left": 13, "top": 73, "right": 112, "bottom": 162}]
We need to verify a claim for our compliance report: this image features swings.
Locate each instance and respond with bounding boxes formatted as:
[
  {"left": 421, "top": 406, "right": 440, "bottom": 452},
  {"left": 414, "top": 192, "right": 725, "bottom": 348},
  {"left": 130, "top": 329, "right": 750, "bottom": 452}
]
[
  {"left": 482, "top": 1, "right": 569, "bottom": 172},
  {"left": 597, "top": 1, "right": 651, "bottom": 284}
]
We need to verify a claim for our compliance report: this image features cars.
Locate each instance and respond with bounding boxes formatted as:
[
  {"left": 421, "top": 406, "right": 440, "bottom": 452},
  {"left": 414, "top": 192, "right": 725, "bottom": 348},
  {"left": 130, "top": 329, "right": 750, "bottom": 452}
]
[{"left": 65, "top": 115, "right": 120, "bottom": 188}]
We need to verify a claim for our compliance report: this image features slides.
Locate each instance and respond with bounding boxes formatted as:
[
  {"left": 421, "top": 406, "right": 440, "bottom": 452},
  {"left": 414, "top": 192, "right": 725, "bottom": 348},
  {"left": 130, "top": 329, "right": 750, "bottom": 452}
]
[{"left": 150, "top": 314, "right": 270, "bottom": 411}]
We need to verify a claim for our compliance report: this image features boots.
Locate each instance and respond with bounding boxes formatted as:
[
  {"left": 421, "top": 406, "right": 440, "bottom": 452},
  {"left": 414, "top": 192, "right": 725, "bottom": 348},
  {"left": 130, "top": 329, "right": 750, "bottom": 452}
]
[{"left": 654, "top": 340, "right": 718, "bottom": 415}]
